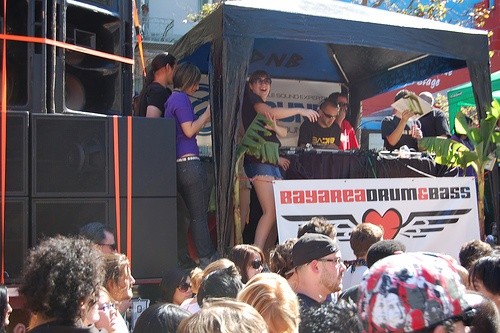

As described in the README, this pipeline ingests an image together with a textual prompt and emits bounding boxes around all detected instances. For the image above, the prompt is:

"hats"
[285,232,339,275]
[357,251,482,333]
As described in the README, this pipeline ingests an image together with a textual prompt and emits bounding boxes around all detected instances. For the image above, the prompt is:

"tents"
[164,0,500,257]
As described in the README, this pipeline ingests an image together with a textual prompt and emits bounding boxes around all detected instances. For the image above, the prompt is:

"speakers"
[0,0,178,283]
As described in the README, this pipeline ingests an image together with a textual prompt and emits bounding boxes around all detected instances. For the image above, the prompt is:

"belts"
[176,155,201,162]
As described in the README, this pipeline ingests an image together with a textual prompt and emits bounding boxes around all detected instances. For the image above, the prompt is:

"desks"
[242,146,436,245]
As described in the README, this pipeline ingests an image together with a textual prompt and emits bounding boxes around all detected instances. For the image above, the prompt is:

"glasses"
[251,259,262,268]
[97,242,118,251]
[336,102,350,107]
[316,255,342,265]
[254,78,272,84]
[322,108,339,119]
[446,308,476,325]
[98,302,116,311]
[177,282,190,292]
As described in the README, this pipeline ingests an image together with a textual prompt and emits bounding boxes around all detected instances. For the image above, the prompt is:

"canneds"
[411,125,418,138]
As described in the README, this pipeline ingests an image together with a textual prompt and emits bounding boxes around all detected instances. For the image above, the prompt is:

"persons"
[298,101,344,150]
[447,116,478,186]
[417,92,453,138]
[380,89,426,150]
[243,71,320,259]
[325,92,359,150]
[0,218,500,333]
[164,62,216,265]
[138,53,179,118]
